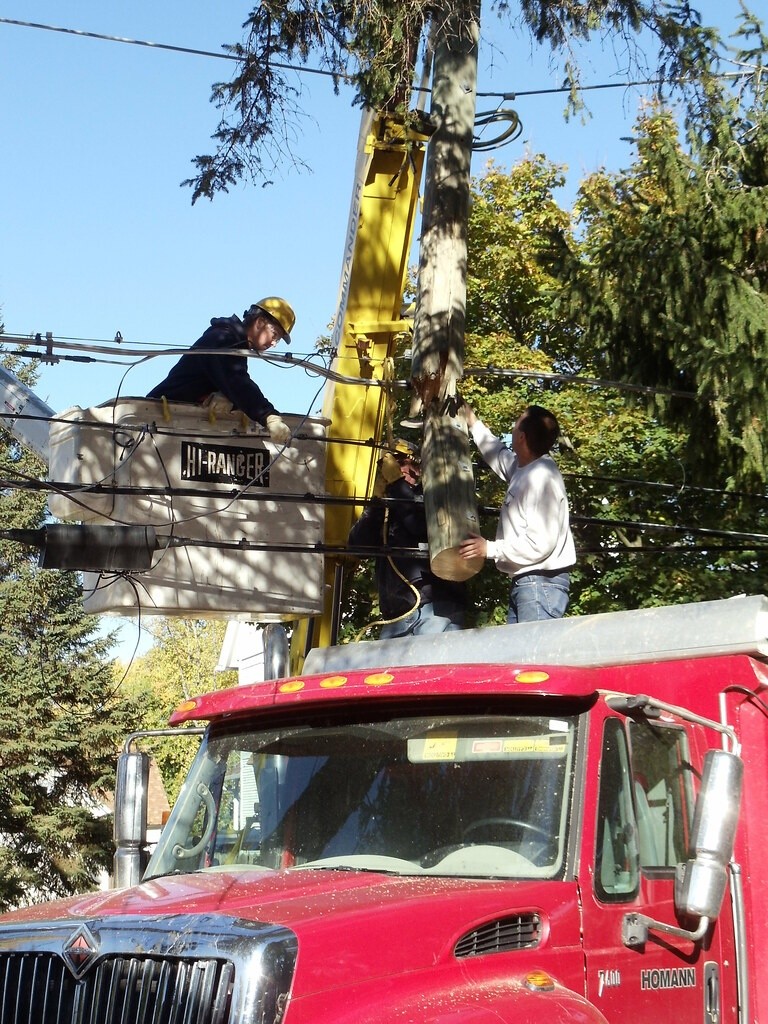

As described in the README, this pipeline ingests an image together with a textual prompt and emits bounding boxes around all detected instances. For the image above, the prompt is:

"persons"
[145,296,296,445]
[346,438,467,640]
[459,401,577,624]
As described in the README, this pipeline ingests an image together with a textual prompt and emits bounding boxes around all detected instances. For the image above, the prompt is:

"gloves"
[267,414,292,448]
[373,473,389,503]
[382,453,406,484]
[203,390,233,424]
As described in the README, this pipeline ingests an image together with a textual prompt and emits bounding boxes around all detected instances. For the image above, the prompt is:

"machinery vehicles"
[0,0,768,1024]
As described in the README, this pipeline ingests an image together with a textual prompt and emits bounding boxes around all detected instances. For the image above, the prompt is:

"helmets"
[251,296,295,344]
[377,438,420,466]
